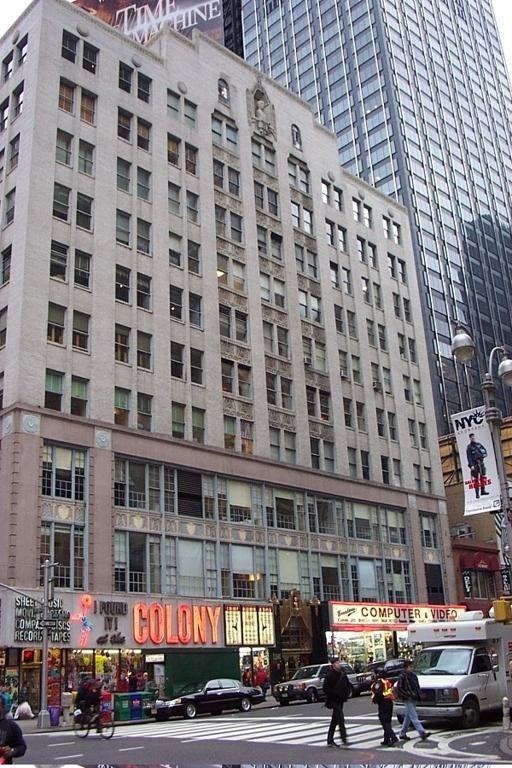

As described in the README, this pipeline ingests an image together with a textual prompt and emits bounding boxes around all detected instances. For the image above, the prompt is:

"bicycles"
[72,703,116,741]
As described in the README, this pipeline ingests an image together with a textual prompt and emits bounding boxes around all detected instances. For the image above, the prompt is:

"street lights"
[449,321,512,599]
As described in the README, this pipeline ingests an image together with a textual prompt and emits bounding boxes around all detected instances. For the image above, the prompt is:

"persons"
[322,657,353,746]
[0,680,15,720]
[243,667,252,686]
[467,432,489,498]
[397,661,431,741]
[257,668,268,695]
[0,694,27,764]
[75,672,104,732]
[371,664,400,747]
[13,678,37,719]
[116,669,149,691]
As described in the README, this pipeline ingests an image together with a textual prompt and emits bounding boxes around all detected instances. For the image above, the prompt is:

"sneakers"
[399,735,411,741]
[341,738,352,745]
[380,740,391,745]
[422,732,432,740]
[327,741,340,749]
[388,738,398,747]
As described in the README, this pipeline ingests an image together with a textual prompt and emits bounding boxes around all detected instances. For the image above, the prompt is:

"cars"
[143,677,266,720]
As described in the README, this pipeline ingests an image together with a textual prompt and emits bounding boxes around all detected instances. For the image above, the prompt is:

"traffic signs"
[35,618,59,627]
[51,613,70,621]
[32,608,44,614]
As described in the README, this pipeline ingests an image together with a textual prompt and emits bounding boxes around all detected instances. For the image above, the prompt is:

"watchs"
[10,746,18,756]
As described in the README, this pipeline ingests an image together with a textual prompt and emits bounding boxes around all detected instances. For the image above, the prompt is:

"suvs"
[356,656,412,694]
[271,661,360,706]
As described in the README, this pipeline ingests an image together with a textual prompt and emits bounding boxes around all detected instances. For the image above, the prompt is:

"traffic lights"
[23,650,36,662]
[493,595,512,624]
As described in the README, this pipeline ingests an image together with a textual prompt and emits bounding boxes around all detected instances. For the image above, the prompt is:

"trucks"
[391,610,512,729]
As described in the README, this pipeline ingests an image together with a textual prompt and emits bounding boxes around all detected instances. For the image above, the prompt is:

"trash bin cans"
[47,705,61,727]
[100,691,153,723]
